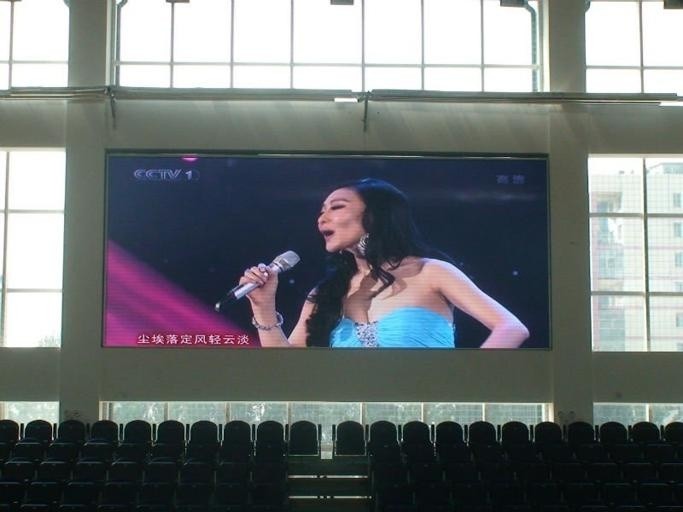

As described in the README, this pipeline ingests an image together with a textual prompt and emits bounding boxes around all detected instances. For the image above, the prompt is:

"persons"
[238,178,530,350]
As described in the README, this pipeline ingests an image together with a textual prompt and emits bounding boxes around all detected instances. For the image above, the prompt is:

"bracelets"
[250,310,285,331]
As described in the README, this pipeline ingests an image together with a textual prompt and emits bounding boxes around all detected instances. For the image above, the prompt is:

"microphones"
[215,250,301,313]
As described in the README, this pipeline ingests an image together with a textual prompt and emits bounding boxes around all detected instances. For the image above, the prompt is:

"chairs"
[1,416,288,510]
[288,420,322,500]
[369,421,683,511]
[331,420,369,497]
